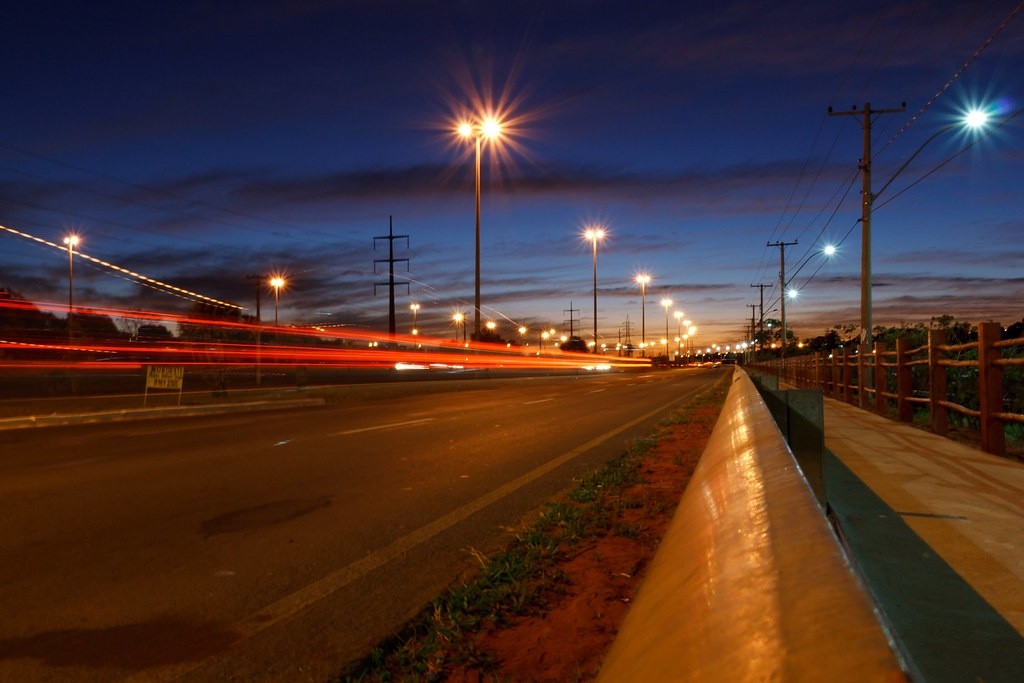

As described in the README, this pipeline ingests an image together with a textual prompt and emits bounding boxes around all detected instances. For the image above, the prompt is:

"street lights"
[457,113,503,342]
[410,303,421,350]
[269,277,285,345]
[760,288,799,361]
[857,108,991,402]
[453,313,463,340]
[675,311,685,367]
[661,298,672,364]
[62,234,79,340]
[751,308,779,362]
[584,227,604,354]
[636,273,650,359]
[778,244,837,383]
